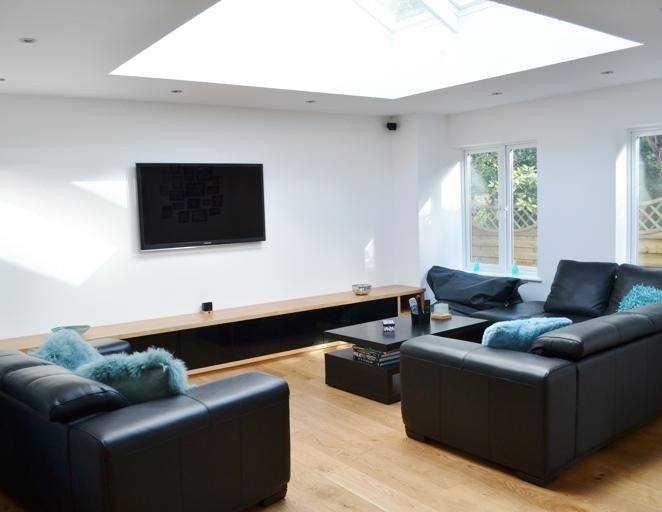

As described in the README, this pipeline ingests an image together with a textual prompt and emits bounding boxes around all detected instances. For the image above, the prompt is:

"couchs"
[400,264,661,486]
[0,339,290,512]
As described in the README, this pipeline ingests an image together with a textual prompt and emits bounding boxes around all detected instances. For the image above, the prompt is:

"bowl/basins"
[351,284,372,296]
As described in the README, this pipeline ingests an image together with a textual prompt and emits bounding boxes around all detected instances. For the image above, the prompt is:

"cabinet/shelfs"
[0,284,427,378]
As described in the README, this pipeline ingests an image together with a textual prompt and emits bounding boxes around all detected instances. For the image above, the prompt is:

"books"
[352,344,400,367]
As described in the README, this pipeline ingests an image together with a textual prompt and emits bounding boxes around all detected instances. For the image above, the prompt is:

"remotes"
[424,300,429,313]
[409,295,418,315]
[416,295,423,314]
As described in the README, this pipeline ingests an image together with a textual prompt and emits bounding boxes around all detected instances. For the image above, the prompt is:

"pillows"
[31,327,104,377]
[615,282,661,312]
[541,255,619,315]
[481,316,572,349]
[70,347,197,403]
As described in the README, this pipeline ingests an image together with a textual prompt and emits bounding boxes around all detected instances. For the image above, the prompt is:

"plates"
[51,326,89,335]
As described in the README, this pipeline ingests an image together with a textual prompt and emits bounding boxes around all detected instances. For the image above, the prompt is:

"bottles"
[473,257,480,271]
[512,261,519,276]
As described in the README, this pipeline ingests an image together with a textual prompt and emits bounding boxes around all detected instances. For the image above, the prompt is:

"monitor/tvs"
[136,163,265,250]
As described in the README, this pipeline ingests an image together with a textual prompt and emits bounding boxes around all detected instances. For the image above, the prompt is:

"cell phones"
[382,319,395,326]
